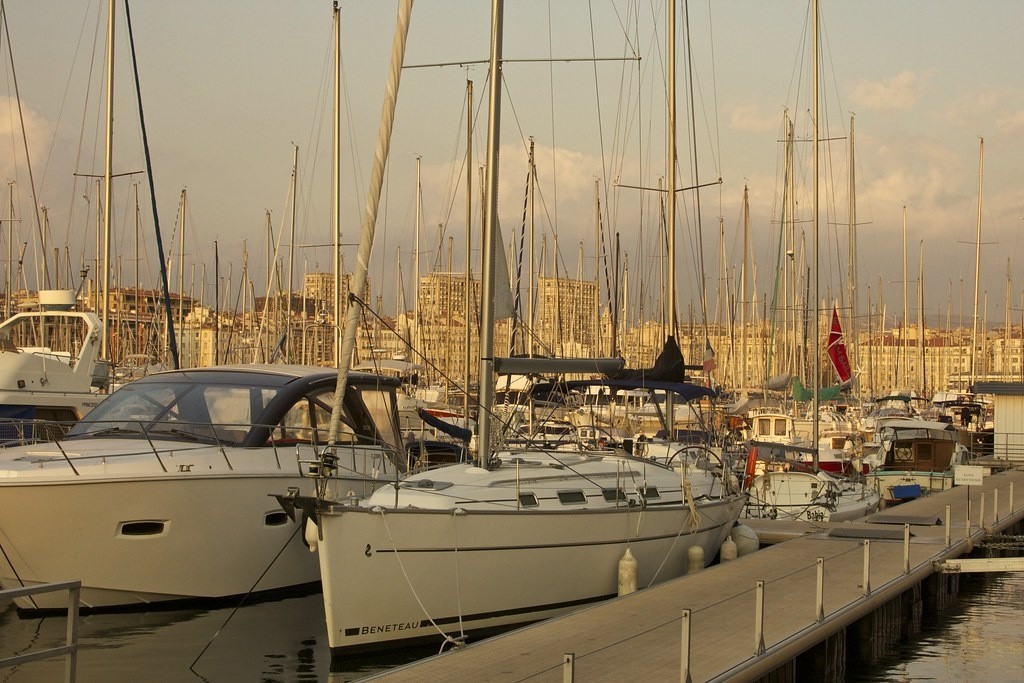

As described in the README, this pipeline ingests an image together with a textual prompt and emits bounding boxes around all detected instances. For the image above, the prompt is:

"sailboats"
[0,0,1021,674]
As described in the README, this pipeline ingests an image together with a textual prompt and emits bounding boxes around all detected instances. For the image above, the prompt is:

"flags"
[703,332,718,374]
[825,303,853,384]
